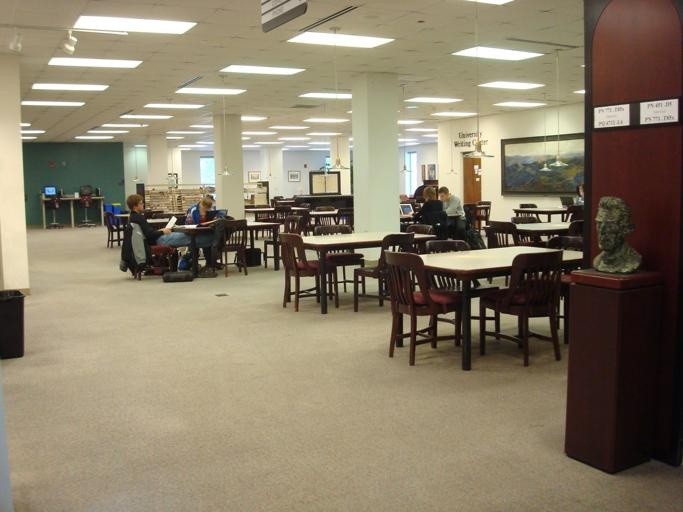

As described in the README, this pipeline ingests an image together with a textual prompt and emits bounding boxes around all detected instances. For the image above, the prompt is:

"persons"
[127,194,192,248]
[412,187,444,226]
[594,195,643,273]
[178,195,226,271]
[438,185,466,239]
[400,183,426,215]
[574,184,584,205]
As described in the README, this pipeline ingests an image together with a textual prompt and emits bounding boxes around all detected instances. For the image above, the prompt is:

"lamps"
[399,83,410,173]
[129,147,140,182]
[1,23,129,57]
[215,96,239,177]
[461,3,499,158]
[168,146,177,187]
[538,47,570,173]
[329,25,351,171]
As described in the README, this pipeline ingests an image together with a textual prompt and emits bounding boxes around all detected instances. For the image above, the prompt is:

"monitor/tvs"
[44,185,58,197]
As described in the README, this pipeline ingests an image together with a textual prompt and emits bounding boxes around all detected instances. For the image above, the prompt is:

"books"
[164,216,178,229]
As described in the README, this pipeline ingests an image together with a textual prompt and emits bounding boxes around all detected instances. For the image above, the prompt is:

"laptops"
[204,209,228,224]
[400,202,414,216]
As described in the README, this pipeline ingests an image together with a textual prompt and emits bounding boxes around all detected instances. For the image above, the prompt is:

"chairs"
[47,196,62,228]
[79,194,97,227]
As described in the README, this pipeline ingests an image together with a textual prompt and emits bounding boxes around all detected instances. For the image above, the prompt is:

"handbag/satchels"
[236,248,262,268]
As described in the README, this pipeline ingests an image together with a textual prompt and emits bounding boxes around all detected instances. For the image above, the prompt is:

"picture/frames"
[500,132,585,198]
[428,164,436,180]
[289,171,300,182]
[248,172,261,182]
[421,164,426,179]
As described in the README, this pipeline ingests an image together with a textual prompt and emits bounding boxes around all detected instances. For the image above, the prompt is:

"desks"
[38,189,105,229]
[294,194,354,207]
